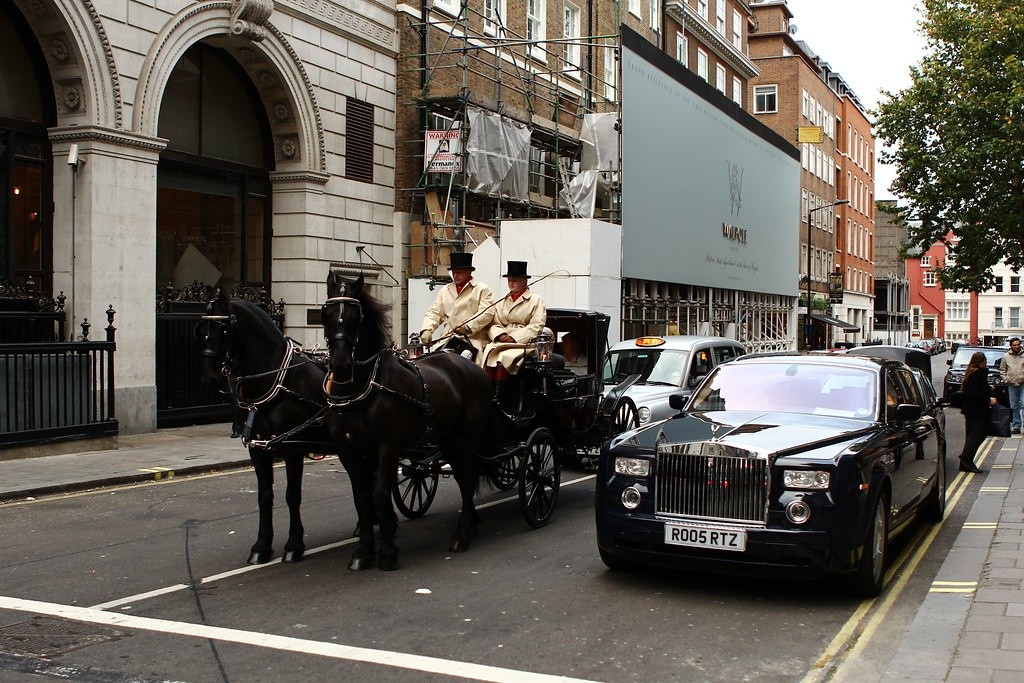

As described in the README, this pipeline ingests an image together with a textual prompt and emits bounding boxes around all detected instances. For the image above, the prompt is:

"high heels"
[959,460,982,473]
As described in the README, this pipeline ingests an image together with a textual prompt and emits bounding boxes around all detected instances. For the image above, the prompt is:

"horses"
[321,270,500,571]
[194,285,379,565]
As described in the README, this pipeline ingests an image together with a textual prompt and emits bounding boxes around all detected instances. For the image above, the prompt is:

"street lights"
[808,200,850,345]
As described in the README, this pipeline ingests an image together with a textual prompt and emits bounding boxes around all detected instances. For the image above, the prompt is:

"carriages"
[196,272,640,571]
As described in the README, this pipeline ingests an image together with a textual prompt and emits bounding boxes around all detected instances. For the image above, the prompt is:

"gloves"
[420,330,432,346]
[454,324,466,335]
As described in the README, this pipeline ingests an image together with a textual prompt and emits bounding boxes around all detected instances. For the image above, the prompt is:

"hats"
[503,261,531,278]
[447,253,475,270]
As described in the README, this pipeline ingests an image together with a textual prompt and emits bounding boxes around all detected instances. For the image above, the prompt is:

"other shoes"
[1012,429,1020,434]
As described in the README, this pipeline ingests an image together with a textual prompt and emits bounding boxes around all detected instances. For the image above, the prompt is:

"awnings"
[810,314,861,333]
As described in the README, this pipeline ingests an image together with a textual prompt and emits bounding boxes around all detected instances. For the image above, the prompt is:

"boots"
[491,381,505,405]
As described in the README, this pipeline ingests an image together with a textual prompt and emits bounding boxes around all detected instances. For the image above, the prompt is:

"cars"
[553,335,747,467]
[595,345,947,598]
[944,346,1010,404]
[951,339,970,354]
[905,337,947,356]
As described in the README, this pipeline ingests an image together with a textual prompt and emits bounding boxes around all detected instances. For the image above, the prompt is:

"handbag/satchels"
[987,404,1011,437]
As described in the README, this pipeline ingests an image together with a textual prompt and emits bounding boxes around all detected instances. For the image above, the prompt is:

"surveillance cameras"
[68,144,79,166]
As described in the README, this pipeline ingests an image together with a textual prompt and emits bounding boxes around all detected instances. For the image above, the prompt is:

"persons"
[959,352,998,474]
[539,327,588,376]
[420,252,547,408]
[999,339,1024,434]
